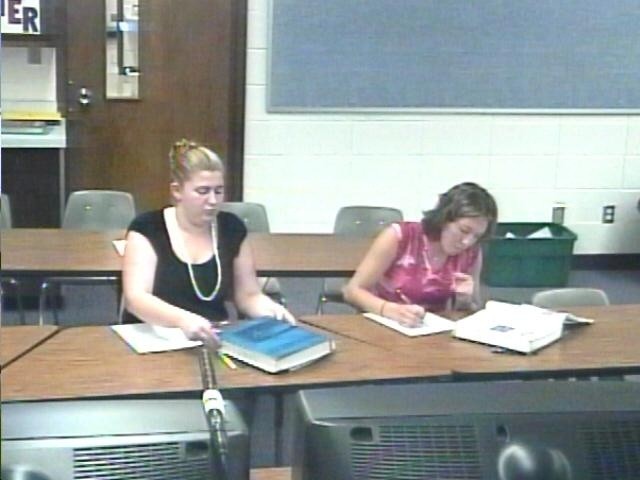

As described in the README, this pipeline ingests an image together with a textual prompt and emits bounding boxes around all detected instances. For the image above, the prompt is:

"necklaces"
[183,219,224,302]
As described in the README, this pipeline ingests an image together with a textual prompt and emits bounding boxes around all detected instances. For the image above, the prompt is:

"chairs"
[221,201,284,308]
[531,288,608,305]
[315,206,403,316]
[38,189,135,326]
[0,194,24,326]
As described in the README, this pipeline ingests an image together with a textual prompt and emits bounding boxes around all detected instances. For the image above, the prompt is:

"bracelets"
[381,300,388,316]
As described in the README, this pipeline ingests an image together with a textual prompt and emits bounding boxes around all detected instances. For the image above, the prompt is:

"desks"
[1,228,374,273]
[0,323,62,370]
[450,305,640,375]
[1,315,449,401]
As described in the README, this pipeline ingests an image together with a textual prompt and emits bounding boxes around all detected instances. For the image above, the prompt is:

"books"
[109,323,204,354]
[1,119,47,135]
[451,300,595,354]
[214,317,334,374]
[362,312,456,337]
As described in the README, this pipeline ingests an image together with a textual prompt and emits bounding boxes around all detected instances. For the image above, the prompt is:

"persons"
[121,139,298,350]
[343,181,498,328]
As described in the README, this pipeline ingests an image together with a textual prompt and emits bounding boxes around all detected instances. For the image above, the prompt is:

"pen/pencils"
[218,351,237,368]
[395,289,427,321]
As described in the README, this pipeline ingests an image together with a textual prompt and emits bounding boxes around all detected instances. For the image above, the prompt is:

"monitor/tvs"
[0,398,250,480]
[291,381,639,480]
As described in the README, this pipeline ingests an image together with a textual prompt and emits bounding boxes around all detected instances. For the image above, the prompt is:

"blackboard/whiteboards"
[264,0,639,112]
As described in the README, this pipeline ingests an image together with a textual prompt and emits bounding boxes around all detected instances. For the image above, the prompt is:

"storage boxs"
[483,222,577,286]
